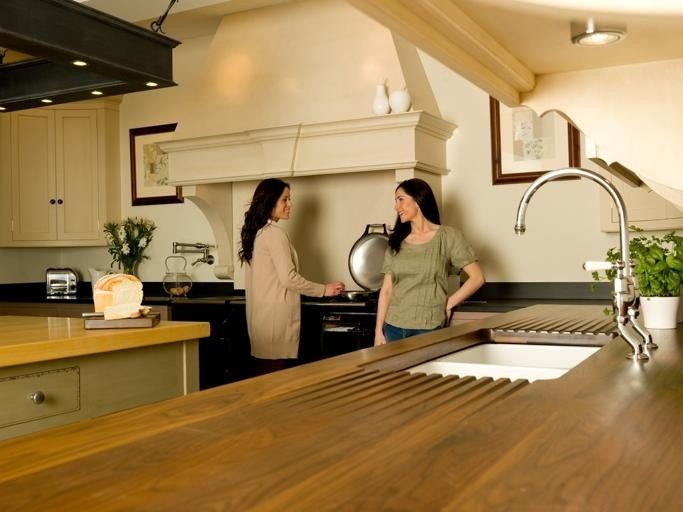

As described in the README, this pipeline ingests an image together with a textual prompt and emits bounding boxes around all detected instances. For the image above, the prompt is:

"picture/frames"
[128,123,188,207]
[487,88,583,186]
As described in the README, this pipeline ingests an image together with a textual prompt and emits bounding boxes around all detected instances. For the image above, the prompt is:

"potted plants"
[585,227,682,330]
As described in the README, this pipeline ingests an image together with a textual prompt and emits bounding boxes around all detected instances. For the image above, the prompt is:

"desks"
[0,310,210,442]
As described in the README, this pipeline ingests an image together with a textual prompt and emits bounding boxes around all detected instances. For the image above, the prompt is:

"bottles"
[372,83,412,114]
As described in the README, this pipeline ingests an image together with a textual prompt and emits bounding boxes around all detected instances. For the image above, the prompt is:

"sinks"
[357,326,617,380]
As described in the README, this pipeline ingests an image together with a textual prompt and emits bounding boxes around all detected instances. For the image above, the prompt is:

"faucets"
[514,167,658,361]
[172,242,216,264]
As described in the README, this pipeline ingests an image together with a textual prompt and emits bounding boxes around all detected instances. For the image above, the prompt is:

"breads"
[92,274,151,320]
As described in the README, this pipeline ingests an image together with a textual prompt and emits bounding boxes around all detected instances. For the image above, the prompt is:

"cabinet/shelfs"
[174,304,318,389]
[0,96,120,250]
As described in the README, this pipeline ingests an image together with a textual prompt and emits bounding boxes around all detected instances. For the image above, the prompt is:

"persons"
[372,178,486,348]
[237,178,345,378]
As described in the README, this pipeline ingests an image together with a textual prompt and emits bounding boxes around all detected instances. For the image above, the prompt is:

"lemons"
[170,286,190,295]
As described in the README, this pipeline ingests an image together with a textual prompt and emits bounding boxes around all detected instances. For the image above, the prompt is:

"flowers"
[96,213,157,275]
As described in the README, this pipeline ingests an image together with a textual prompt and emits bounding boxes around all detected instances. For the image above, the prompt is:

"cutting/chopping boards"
[84,311,162,330]
